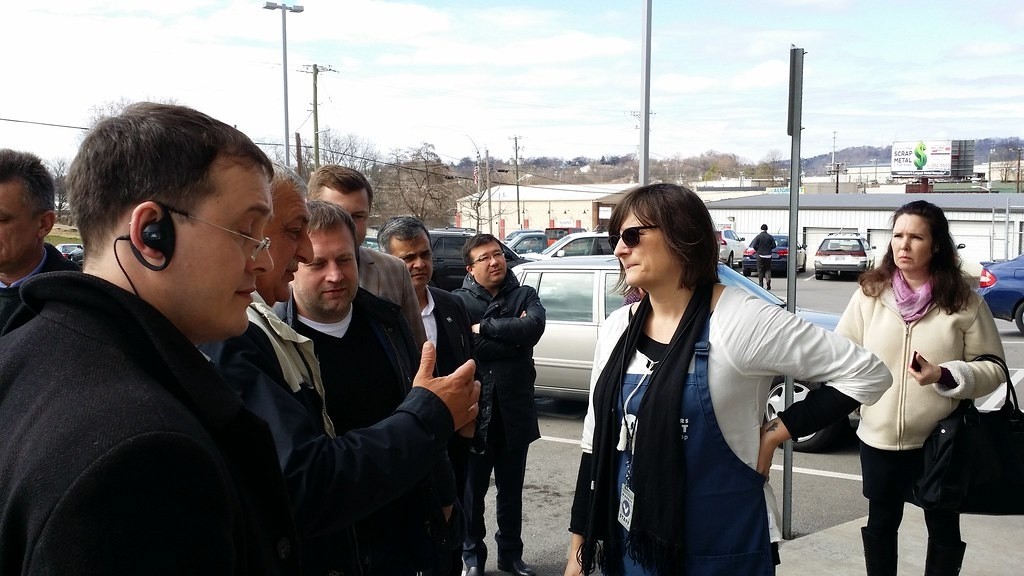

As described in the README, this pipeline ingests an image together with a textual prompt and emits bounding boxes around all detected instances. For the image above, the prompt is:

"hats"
[761,223,768,230]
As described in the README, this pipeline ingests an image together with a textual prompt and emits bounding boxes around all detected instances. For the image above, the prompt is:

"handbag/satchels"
[911,354,1024,515]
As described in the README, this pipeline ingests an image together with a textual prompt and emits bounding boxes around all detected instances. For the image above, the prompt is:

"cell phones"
[910,351,927,372]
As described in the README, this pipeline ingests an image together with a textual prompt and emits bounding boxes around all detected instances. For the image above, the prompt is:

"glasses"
[607,225,659,248]
[128,201,272,259]
[469,250,505,266]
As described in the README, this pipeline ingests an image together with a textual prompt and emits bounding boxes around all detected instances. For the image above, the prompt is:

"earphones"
[113,199,178,299]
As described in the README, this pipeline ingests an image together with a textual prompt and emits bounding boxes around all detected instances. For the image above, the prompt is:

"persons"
[189,157,483,576]
[562,185,893,576]
[267,200,469,576]
[304,165,439,384]
[446,231,547,576]
[754,225,777,290]
[378,214,476,511]
[0,148,85,331]
[1,100,304,574]
[831,200,1008,576]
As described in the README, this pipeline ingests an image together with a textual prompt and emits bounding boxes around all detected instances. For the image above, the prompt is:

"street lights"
[263,2,304,166]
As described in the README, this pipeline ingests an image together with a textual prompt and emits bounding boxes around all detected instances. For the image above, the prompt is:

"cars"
[948,230,965,268]
[511,255,858,453]
[716,229,748,270]
[976,252,1024,333]
[56,243,85,270]
[742,226,807,278]
[814,227,876,281]
[355,225,613,295]
